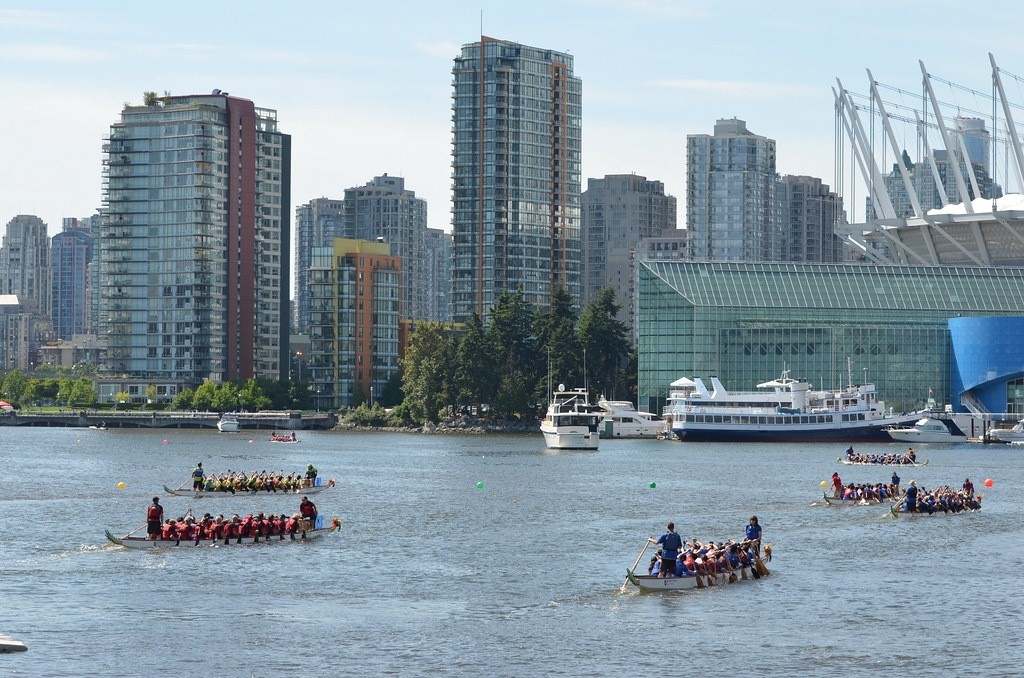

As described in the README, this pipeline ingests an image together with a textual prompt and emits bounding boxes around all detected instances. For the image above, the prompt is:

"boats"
[270,438,301,444]
[217,414,240,432]
[662,357,1024,445]
[823,492,981,517]
[837,457,928,467]
[162,478,335,496]
[89,426,109,431]
[627,544,771,590]
[105,518,342,547]
[540,347,680,448]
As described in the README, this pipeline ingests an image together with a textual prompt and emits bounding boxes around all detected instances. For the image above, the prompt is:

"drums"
[301,479,310,489]
[299,518,308,532]
[850,454,855,461]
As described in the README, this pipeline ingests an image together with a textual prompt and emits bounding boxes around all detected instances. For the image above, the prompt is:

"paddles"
[843,482,897,506]
[167,476,193,494]
[683,537,770,589]
[212,469,296,494]
[905,455,918,467]
[887,496,904,514]
[816,488,832,502]
[167,508,308,546]
[620,534,652,589]
[105,522,148,546]
[852,454,904,466]
[917,484,981,515]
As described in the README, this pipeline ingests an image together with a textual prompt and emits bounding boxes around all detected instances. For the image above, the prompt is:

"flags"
[929,388,933,394]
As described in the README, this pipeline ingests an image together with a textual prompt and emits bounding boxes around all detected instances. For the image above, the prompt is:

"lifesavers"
[842,405,847,411]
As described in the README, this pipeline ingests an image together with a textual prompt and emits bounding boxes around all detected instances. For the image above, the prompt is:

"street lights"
[369,386,374,407]
[83,338,91,374]
[5,392,15,404]
[297,351,302,381]
[317,390,321,413]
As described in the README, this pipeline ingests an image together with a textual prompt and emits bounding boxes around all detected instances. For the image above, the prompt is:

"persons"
[845,446,915,464]
[194,409,196,417]
[648,514,762,578]
[901,478,974,513]
[271,431,296,441]
[832,471,900,500]
[147,496,318,541]
[102,421,106,426]
[192,462,317,494]
